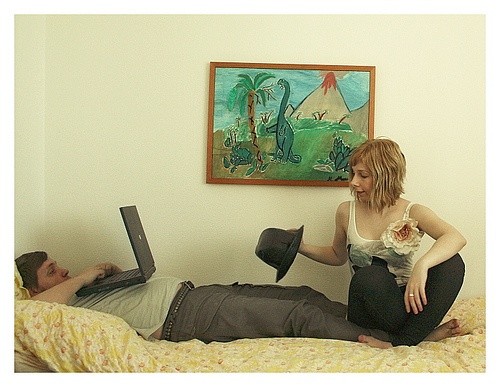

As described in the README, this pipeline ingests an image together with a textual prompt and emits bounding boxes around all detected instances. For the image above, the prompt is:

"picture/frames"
[205,60,376,188]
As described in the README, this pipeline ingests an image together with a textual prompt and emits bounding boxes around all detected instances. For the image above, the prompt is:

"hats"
[254,223,304,284]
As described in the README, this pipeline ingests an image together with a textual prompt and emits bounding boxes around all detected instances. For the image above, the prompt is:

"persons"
[13,251,394,345]
[286,137,467,350]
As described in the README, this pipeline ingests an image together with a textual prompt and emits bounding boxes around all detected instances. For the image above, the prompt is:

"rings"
[408,294,414,296]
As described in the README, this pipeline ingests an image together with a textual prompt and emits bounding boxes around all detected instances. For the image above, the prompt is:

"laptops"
[75,206,156,296]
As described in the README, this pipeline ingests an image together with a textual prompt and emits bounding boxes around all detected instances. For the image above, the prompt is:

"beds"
[13,260,484,371]
